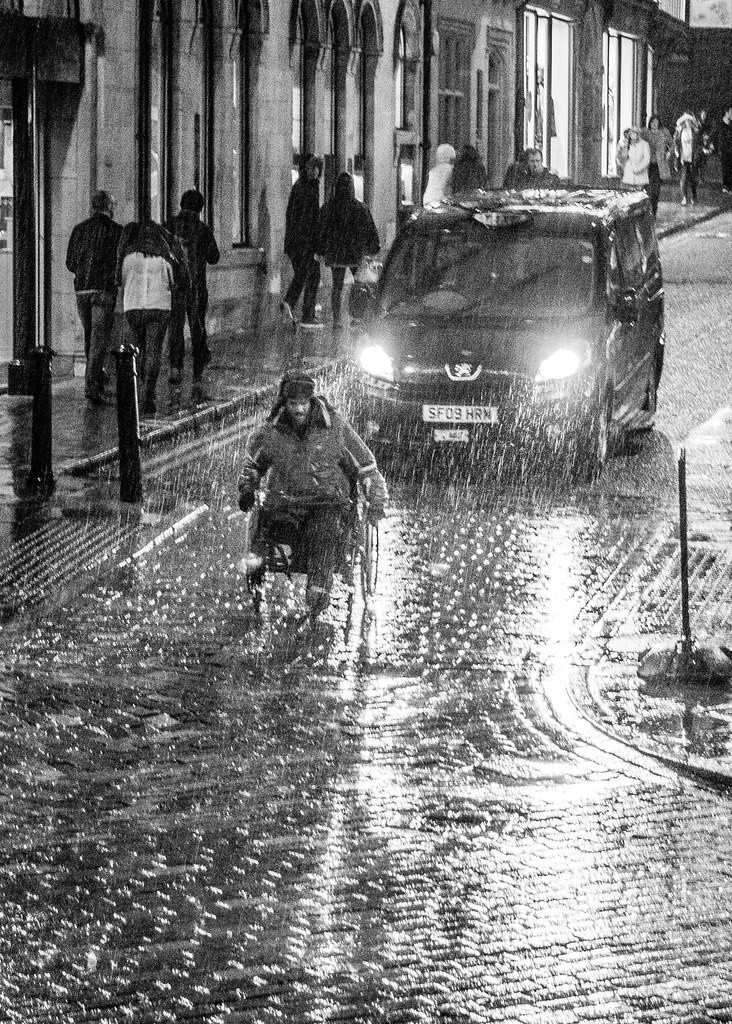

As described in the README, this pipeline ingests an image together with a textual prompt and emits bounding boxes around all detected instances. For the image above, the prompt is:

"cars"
[346,185,666,487]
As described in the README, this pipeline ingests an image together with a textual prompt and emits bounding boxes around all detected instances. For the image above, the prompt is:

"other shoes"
[281,302,292,322]
[143,396,155,413]
[309,589,331,613]
[86,390,103,403]
[300,319,323,329]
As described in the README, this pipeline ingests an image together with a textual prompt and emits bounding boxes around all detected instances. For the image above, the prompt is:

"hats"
[267,370,331,429]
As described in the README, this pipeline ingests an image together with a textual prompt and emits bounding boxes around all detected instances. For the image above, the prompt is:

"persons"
[63,189,122,406]
[672,104,732,207]
[161,190,221,401]
[115,218,193,412]
[279,150,328,329]
[315,169,381,332]
[616,114,678,223]
[502,148,561,189]
[238,371,387,611]
[420,142,486,210]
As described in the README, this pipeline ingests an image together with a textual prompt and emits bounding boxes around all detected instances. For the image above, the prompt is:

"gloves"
[239,492,255,512]
[366,504,384,525]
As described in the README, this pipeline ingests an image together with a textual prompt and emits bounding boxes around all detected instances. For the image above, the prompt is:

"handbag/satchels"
[349,259,377,317]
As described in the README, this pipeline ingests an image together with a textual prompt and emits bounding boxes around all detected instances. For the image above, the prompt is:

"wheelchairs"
[242,489,381,607]
